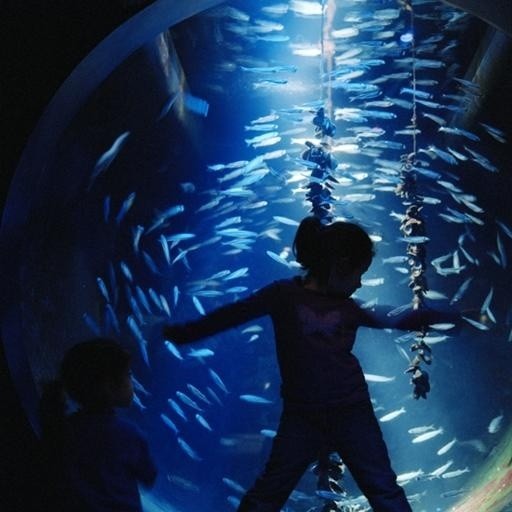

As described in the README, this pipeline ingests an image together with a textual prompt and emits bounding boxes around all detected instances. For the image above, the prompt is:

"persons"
[157,217,484,512]
[43,339,154,512]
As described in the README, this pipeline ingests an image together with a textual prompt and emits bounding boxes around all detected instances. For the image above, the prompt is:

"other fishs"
[83,0,512,512]
